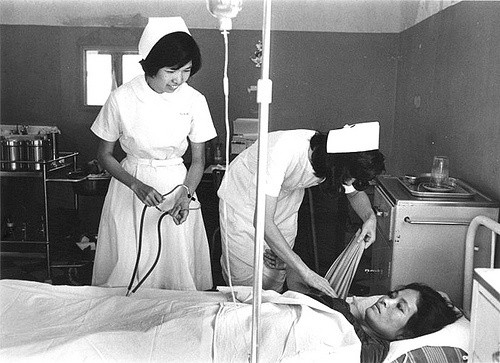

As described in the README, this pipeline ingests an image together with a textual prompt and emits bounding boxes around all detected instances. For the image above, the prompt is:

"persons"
[91,17,217,293]
[218,121,386,296]
[302,282,456,363]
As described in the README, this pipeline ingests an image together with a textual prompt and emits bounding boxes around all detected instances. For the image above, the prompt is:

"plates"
[423,183,456,192]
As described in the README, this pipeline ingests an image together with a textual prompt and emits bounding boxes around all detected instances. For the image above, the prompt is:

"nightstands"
[468,268,500,363]
[369,177,499,309]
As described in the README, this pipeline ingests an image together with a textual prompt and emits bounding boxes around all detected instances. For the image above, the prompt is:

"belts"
[128,155,184,167]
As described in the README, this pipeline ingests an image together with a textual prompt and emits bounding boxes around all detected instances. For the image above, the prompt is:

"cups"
[430,155,449,189]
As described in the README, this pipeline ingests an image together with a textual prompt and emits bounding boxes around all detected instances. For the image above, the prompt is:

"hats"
[138,16,192,60]
[327,121,380,153]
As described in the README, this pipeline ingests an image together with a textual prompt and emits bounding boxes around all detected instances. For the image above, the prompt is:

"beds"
[0,215,500,363]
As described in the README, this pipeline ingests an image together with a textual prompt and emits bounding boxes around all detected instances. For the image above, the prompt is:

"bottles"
[204,135,224,165]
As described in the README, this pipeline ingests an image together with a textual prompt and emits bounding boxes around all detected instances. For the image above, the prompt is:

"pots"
[0,135,51,171]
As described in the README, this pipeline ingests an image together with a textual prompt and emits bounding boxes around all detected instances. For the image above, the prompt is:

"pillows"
[351,295,469,363]
